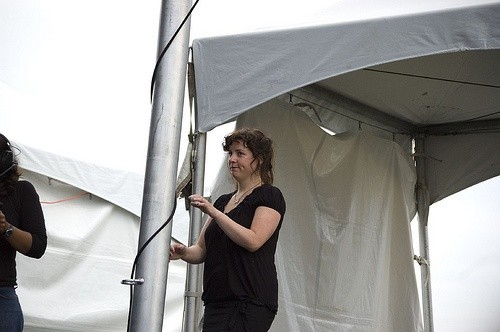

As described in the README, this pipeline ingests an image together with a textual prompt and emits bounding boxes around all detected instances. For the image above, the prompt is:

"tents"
[0,138,192,332]
[186,1,500,331]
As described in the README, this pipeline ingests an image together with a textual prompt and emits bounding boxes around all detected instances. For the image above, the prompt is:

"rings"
[202,197,205,203]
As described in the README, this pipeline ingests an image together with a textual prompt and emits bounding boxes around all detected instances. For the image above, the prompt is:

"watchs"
[2,223,14,237]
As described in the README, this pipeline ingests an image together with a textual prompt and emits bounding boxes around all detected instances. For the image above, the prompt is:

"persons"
[0,133,48,332]
[168,127,287,332]
